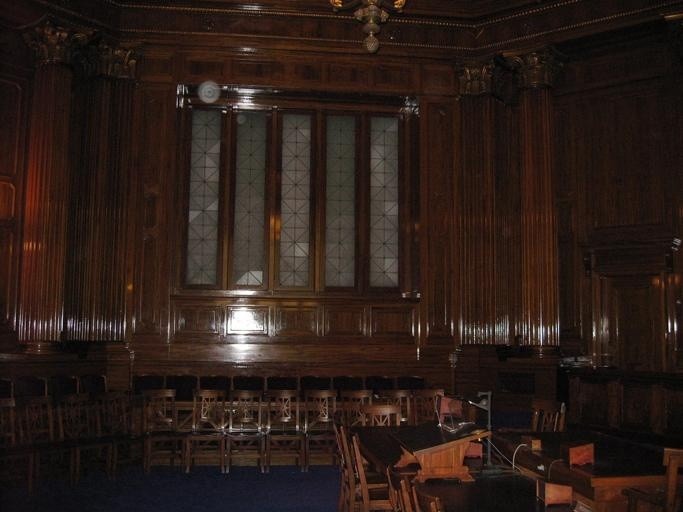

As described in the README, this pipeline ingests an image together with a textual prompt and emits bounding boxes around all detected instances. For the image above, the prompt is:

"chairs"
[334,390,683,512]
[186,391,226,474]
[305,390,338,470]
[146,391,186,474]
[266,391,304,472]
[226,391,265,473]
[2,390,146,494]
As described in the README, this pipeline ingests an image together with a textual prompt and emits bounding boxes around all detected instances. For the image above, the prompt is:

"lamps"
[331,0,407,53]
